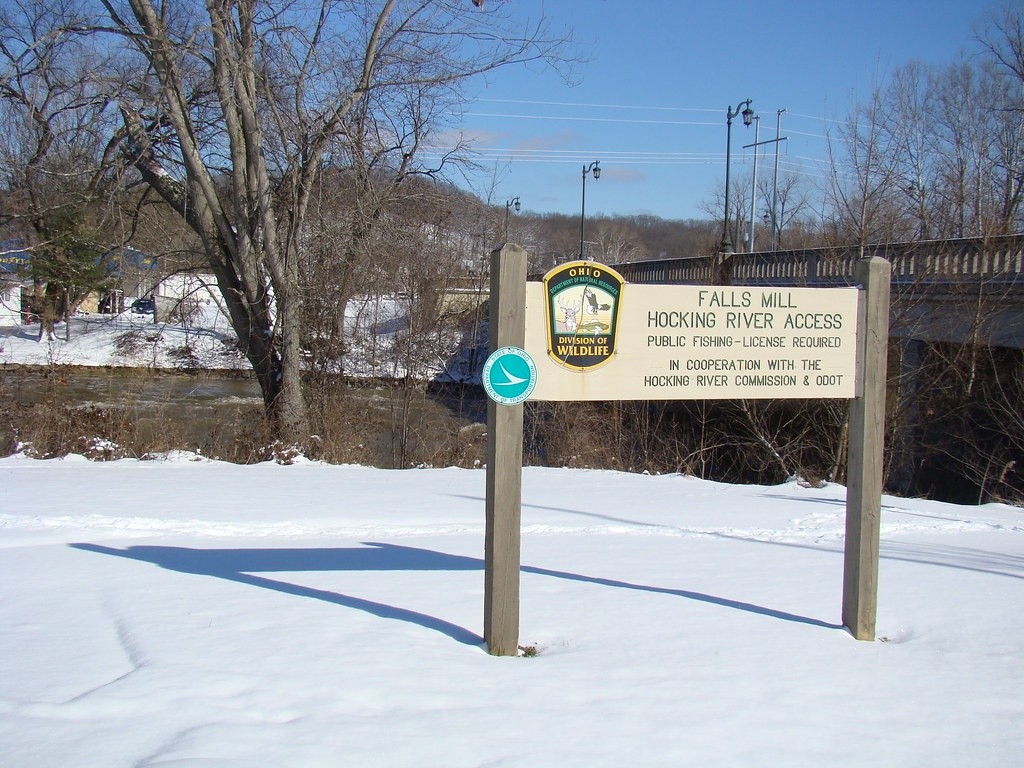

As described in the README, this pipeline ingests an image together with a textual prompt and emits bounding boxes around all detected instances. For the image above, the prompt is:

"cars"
[131,298,154,314]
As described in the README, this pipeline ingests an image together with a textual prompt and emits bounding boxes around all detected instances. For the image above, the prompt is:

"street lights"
[720,99,754,253]
[506,196,521,243]
[579,160,601,260]
